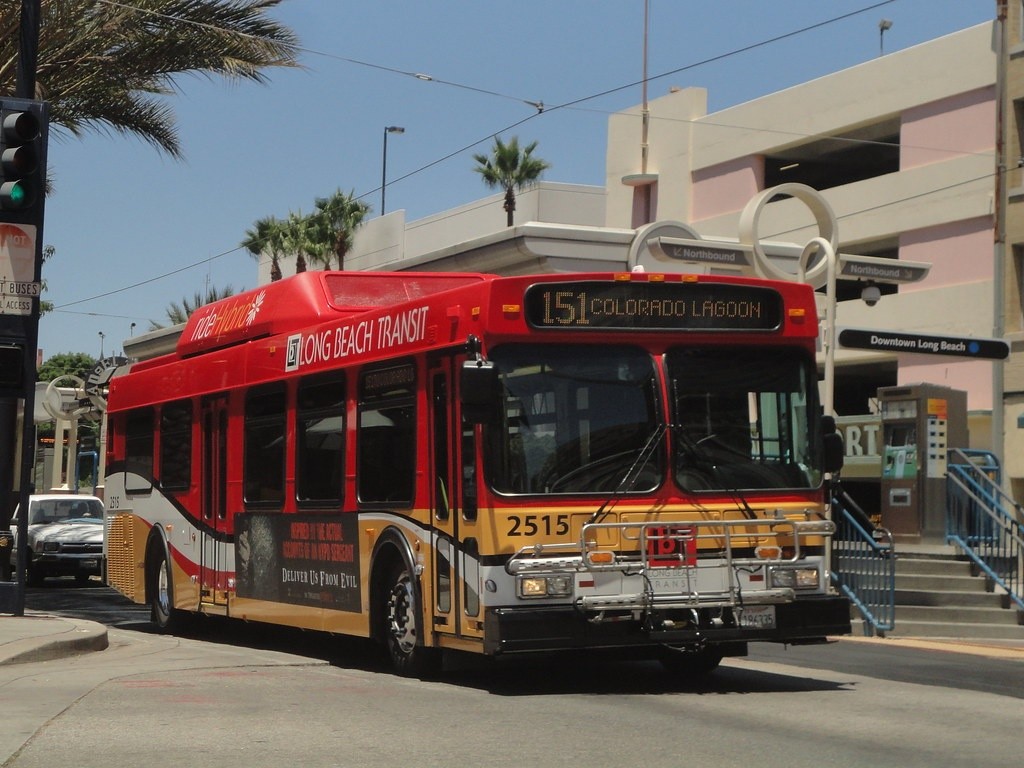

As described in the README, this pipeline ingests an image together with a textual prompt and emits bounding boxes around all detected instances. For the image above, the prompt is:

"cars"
[9,492,105,586]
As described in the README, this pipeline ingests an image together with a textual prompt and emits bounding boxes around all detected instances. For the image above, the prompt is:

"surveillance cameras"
[861,287,881,306]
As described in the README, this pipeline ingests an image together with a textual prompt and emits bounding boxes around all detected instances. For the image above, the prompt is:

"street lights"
[381,125,404,214]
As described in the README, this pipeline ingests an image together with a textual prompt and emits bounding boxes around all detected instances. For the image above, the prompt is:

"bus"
[97,269,854,683]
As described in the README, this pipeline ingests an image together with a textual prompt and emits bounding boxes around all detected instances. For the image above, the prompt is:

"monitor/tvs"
[891,429,908,447]
